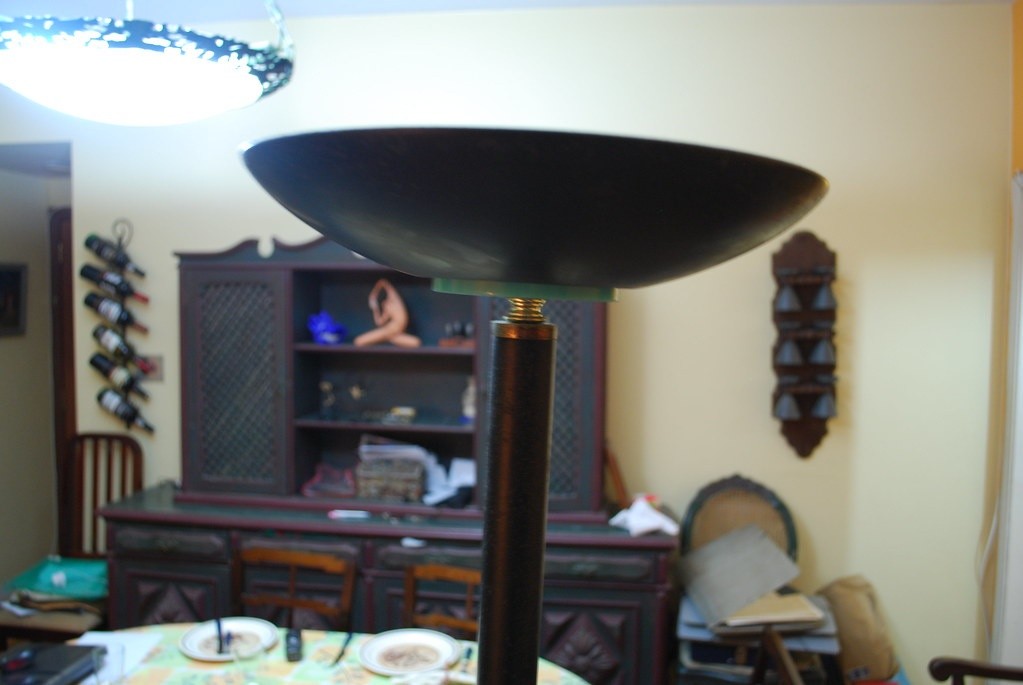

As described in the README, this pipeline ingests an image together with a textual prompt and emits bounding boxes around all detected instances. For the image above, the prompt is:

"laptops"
[0,641,108,685]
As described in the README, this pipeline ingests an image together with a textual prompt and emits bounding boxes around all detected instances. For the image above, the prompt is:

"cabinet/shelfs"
[105,232,676,685]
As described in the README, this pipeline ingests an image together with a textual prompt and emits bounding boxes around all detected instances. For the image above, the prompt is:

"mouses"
[0,647,37,674]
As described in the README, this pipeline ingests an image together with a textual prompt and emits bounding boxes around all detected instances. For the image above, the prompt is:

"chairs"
[403,563,481,641]
[229,530,358,632]
[1,431,145,655]
[677,472,833,683]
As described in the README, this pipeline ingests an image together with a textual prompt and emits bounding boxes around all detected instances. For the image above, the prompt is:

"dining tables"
[69,618,588,685]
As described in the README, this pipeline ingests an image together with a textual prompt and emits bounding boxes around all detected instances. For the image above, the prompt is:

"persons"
[353,277,421,348]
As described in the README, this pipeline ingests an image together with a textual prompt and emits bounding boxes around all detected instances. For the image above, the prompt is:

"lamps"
[0,1,294,125]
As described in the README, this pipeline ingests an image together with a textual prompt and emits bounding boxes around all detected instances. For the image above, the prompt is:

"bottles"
[84,235,145,278]
[89,354,149,402]
[92,323,153,373]
[96,388,153,434]
[80,265,149,303]
[84,293,147,333]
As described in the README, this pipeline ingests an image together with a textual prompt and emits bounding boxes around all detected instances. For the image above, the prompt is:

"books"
[676,521,827,636]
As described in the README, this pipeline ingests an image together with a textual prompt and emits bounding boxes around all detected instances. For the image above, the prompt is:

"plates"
[356,627,462,676]
[178,616,280,662]
